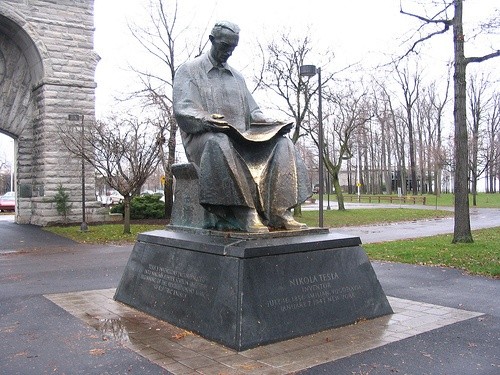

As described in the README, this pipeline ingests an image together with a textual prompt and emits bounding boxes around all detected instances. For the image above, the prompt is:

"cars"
[0,190,15,212]
[104,189,166,204]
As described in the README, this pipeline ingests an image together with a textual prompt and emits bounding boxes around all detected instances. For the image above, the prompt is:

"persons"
[172,21,314,233]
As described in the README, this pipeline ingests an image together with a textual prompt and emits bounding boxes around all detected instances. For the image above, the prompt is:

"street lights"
[67,113,89,230]
[300,64,323,227]
